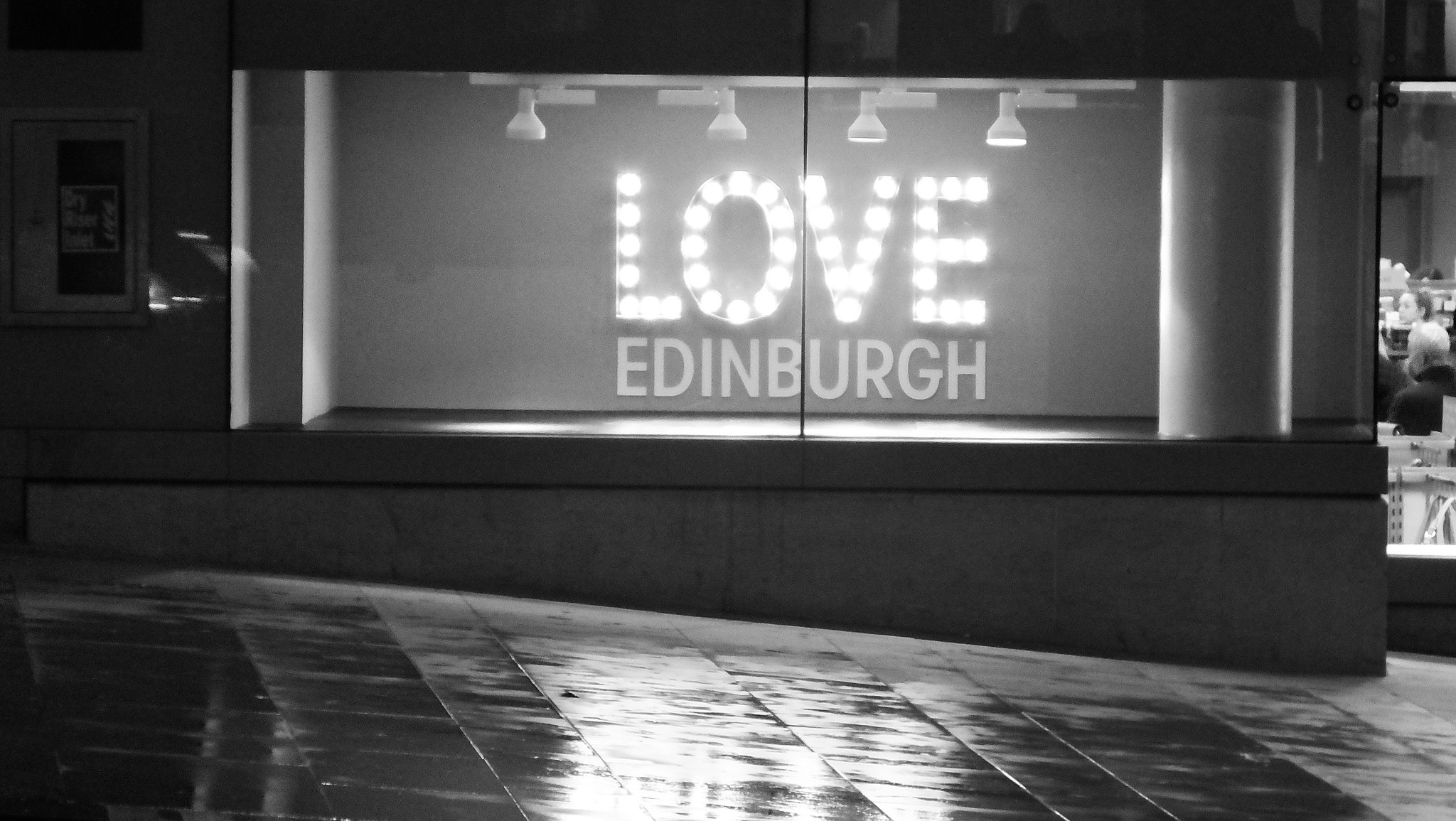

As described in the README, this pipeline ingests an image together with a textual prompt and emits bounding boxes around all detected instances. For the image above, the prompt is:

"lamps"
[847,88,937,143]
[657,87,747,139]
[985,88,1078,147]
[505,85,595,140]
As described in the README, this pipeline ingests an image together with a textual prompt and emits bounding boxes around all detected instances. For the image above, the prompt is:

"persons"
[1387,321,1455,436]
[1377,353,1412,421]
[1410,266,1442,281]
[1397,288,1434,324]
